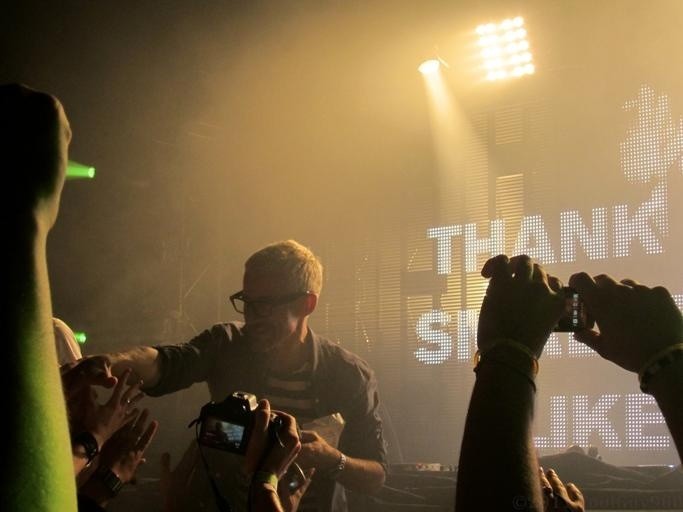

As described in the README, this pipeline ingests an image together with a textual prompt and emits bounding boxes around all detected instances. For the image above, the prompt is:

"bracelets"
[473,340,539,375]
[472,361,536,391]
[639,338,682,394]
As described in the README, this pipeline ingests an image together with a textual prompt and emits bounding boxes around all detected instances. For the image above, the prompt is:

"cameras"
[551,286,596,333]
[197,389,282,457]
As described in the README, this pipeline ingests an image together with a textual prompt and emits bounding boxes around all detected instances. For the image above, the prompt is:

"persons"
[62,239,389,512]
[62,371,314,512]
[540,465,584,511]
[0,84,77,511]
[454,254,681,511]
[53,319,83,364]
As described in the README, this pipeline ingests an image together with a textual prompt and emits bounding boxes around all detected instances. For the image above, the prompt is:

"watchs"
[316,454,346,481]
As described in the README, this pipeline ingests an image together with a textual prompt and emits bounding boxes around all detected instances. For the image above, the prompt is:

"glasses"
[230,289,312,317]
